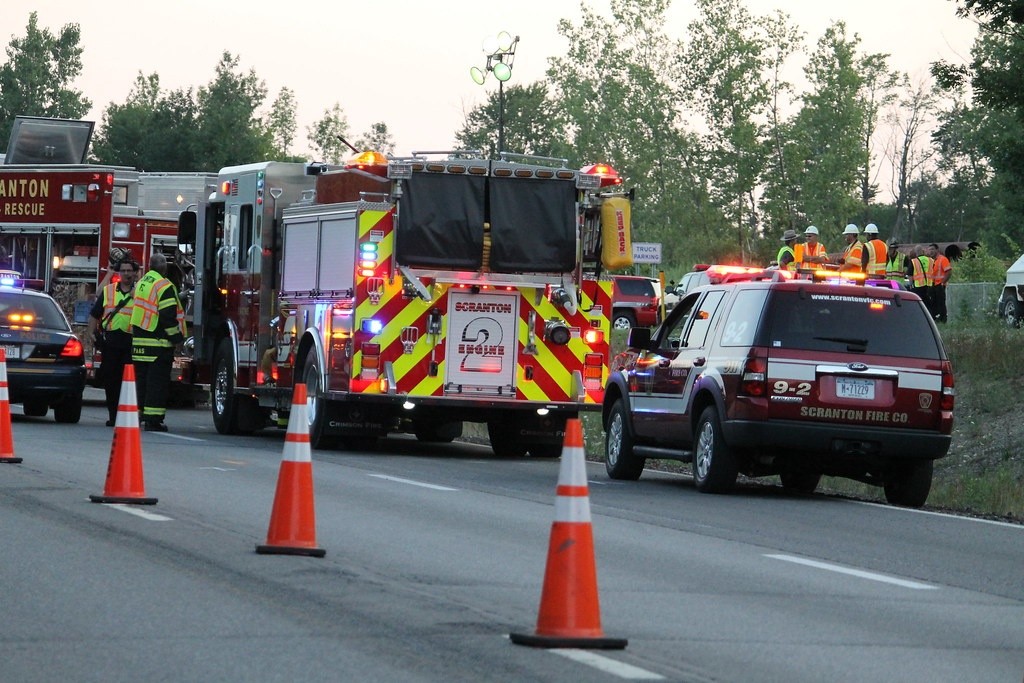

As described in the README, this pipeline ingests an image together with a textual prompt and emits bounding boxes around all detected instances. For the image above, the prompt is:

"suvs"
[600,268,955,508]
[607,274,661,330]
[658,263,742,325]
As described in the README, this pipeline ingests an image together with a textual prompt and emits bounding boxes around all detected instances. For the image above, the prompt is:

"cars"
[998,255,1024,329]
[0,269,86,423]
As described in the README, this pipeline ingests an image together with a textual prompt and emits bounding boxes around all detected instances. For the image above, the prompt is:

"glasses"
[806,234,815,238]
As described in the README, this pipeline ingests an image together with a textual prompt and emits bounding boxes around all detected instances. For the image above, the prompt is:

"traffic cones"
[89,364,159,505]
[255,382,326,558]
[0,349,23,463]
[509,420,629,651]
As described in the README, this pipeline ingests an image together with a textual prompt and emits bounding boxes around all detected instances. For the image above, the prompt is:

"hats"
[780,230,801,241]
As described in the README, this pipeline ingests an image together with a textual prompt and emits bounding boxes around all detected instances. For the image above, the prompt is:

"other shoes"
[144,421,168,431]
[106,408,117,426]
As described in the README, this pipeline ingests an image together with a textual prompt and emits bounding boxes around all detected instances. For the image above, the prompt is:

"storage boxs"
[75,245,91,256]
[91,246,98,256]
[77,284,96,300]
[73,301,95,323]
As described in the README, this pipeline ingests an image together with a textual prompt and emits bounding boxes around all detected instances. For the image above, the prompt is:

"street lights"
[470,32,521,158]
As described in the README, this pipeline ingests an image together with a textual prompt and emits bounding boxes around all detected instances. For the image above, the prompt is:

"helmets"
[863,223,879,233]
[841,224,860,234]
[804,226,819,235]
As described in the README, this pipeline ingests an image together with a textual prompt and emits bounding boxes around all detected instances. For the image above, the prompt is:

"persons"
[777,225,829,270]
[886,243,952,323]
[87,261,137,426]
[838,223,891,279]
[131,254,188,431]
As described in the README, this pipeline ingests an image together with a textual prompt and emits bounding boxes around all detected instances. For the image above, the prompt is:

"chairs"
[811,307,837,335]
[856,304,909,340]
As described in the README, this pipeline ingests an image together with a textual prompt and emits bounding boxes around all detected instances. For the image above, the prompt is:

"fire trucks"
[177,150,636,460]
[0,114,218,410]
